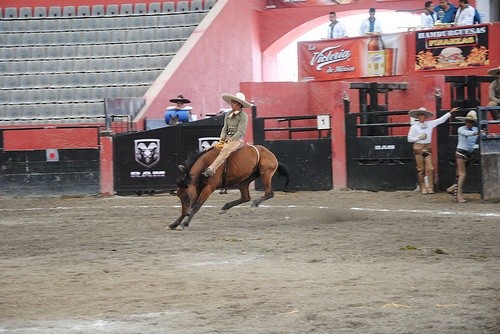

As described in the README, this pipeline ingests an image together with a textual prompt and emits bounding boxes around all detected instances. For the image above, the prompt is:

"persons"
[453,0,480,24]
[360,8,382,35]
[418,1,438,26]
[447,110,485,203]
[166,95,193,111]
[431,0,457,23]
[487,66,500,125]
[325,12,348,38]
[202,92,253,177]
[407,106,459,194]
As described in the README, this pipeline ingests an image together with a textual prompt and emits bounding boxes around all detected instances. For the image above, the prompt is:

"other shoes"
[454,195,467,203]
[446,184,457,192]
[420,188,434,194]
[202,168,214,177]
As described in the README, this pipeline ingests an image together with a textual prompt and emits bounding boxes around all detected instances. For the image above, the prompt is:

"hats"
[487,66,500,74]
[455,110,478,121]
[408,106,434,117]
[169,95,191,104]
[222,92,253,108]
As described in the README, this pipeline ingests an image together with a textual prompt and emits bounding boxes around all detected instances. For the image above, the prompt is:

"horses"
[163,142,292,231]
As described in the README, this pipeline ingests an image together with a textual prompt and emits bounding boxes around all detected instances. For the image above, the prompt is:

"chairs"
[0,0,216,123]
